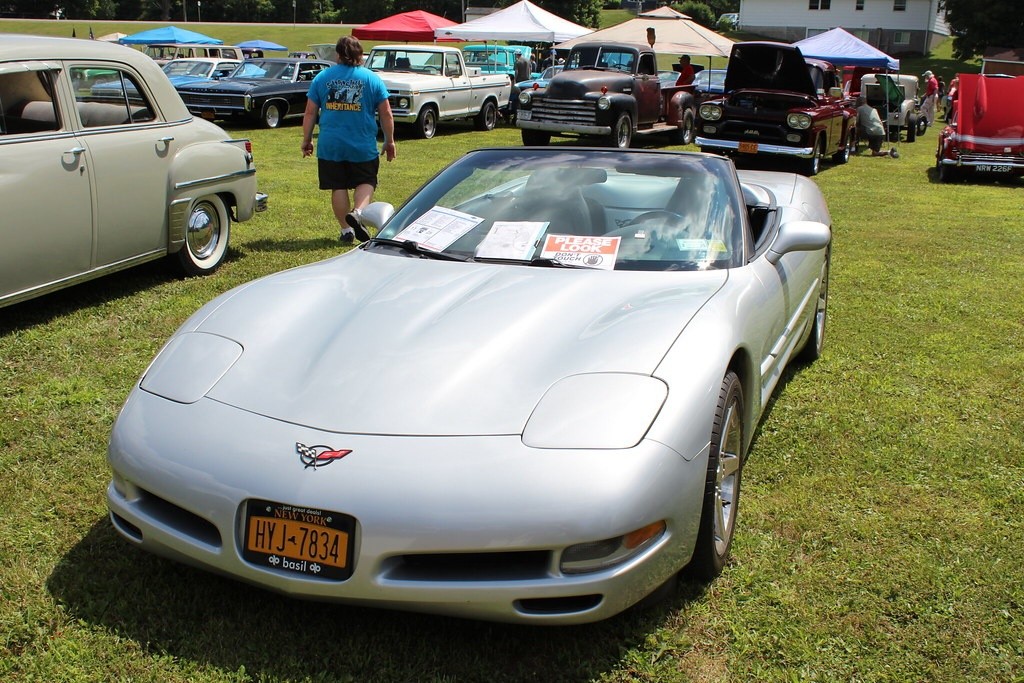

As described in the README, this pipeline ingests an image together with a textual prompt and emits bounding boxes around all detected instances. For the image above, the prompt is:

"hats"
[551,50,557,55]
[922,70,933,76]
[678,55,690,60]
[513,49,522,54]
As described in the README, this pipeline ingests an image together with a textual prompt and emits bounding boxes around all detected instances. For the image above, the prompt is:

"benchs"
[6,99,147,127]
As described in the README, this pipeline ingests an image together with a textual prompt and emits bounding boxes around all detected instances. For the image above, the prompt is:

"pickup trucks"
[364,45,512,139]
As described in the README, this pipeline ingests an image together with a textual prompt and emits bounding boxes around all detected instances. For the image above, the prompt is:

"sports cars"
[107,146,832,625]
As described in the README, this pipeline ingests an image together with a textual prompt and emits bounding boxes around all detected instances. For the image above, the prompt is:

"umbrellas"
[548,5,734,57]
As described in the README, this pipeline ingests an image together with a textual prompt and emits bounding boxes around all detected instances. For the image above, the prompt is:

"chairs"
[519,170,592,238]
[665,177,727,239]
[392,58,411,69]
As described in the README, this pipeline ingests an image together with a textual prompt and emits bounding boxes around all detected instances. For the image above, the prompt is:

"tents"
[232,39,288,51]
[352,9,491,73]
[792,27,900,150]
[96,32,131,43]
[119,25,223,45]
[434,0,596,76]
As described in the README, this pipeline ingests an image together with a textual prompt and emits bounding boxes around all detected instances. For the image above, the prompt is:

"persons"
[541,49,560,73]
[530,54,537,72]
[920,70,959,126]
[854,97,898,158]
[513,50,531,83]
[675,54,696,85]
[300,35,396,243]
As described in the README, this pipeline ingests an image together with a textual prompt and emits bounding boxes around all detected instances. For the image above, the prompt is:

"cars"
[842,66,927,142]
[695,42,860,173]
[0,33,269,309]
[934,59,1024,179]
[463,40,727,148]
[70,43,434,128]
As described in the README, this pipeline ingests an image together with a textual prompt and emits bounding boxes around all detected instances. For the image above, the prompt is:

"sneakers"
[345,209,370,241]
[340,231,354,246]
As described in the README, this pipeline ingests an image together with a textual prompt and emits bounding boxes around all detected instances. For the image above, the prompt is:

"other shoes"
[511,119,516,127]
[890,147,899,158]
[503,119,510,128]
